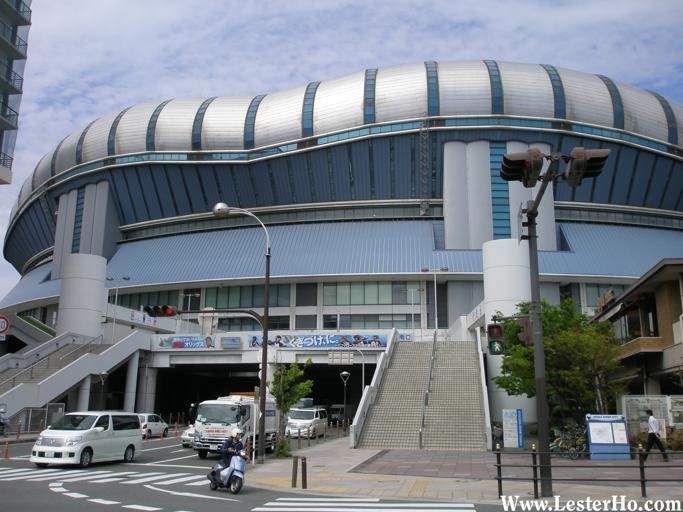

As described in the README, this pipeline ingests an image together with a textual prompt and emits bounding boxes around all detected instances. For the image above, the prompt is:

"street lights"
[104,276,132,347]
[399,287,425,342]
[417,266,449,336]
[338,371,353,438]
[212,199,273,464]
[179,292,201,332]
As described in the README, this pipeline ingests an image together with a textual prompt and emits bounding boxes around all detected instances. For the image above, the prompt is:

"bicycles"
[548,422,590,461]
[491,422,503,447]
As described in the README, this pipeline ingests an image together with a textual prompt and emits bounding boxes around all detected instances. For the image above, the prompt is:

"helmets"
[231,428,242,438]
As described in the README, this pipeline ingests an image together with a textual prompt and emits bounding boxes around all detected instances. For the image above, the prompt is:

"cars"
[180,424,195,448]
[136,411,170,440]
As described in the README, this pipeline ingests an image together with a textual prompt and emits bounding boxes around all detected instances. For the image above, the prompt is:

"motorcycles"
[207,444,256,496]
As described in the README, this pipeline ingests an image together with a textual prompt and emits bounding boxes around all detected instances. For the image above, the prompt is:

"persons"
[205,336,214,348]
[0,409,9,434]
[249,335,287,347]
[643,409,669,462]
[341,335,381,348]
[218,428,244,468]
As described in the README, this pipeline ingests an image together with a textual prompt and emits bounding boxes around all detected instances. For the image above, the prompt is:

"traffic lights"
[486,322,505,356]
[143,304,177,317]
[500,147,543,188]
[566,145,612,190]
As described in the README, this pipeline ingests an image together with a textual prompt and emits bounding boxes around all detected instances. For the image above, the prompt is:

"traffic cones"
[2,440,12,459]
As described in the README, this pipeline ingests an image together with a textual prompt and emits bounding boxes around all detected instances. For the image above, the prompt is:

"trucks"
[188,396,289,460]
[328,403,344,423]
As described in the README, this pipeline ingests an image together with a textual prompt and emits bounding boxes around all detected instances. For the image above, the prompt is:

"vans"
[26,410,144,470]
[286,407,328,439]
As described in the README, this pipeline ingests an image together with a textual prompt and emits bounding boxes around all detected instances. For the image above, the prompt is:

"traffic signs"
[0,314,10,333]
